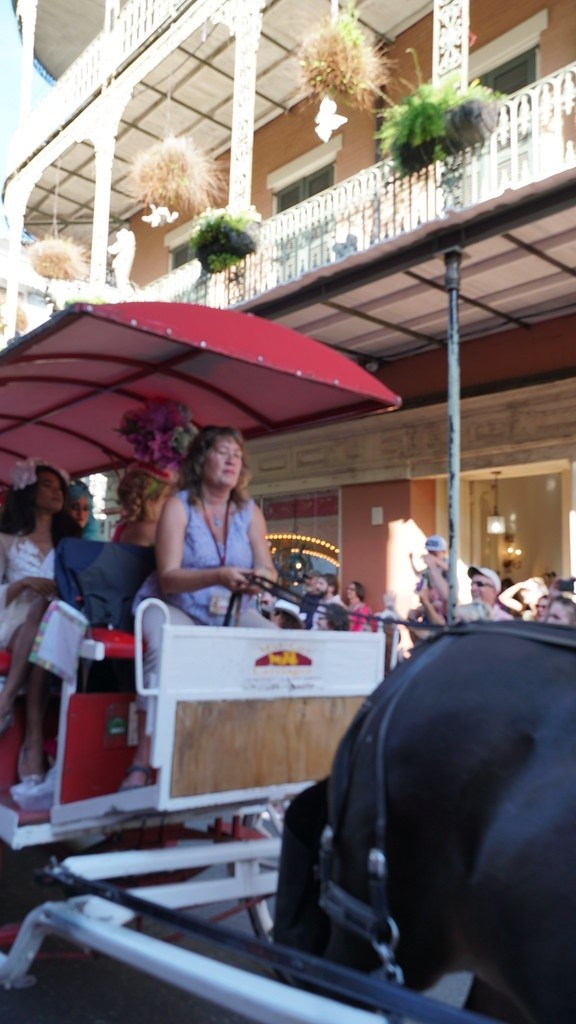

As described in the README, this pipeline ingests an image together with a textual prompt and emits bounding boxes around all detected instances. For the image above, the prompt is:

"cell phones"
[558,580,574,592]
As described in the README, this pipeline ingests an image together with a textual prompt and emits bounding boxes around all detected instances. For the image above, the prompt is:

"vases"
[196,230,256,272]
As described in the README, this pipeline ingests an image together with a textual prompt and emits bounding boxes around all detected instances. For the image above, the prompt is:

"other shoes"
[119,766,150,793]
[17,744,45,785]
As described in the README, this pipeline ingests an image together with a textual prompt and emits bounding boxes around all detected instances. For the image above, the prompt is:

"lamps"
[486,471,506,534]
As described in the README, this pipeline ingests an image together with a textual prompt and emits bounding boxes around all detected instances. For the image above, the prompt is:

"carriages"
[0,297,576,1024]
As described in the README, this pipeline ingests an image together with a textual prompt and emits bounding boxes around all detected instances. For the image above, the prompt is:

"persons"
[108,228,136,287]
[0,458,180,785]
[120,424,279,789]
[267,533,576,671]
[141,204,179,227]
[314,97,337,142]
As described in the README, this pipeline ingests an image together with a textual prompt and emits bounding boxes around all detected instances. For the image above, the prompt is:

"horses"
[276,618,576,1024]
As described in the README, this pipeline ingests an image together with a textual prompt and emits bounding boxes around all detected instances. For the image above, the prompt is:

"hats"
[262,599,307,621]
[468,566,500,593]
[304,569,322,579]
[425,536,447,552]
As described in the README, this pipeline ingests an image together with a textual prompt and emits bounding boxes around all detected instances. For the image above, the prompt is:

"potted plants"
[370,48,507,179]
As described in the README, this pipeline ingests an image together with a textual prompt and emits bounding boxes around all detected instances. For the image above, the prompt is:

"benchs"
[54,540,156,658]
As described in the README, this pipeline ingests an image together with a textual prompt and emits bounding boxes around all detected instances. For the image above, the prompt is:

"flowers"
[185,205,262,273]
[121,400,200,472]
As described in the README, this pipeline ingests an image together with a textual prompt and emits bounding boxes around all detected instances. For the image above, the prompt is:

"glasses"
[535,605,546,608]
[471,581,493,587]
[261,601,268,605]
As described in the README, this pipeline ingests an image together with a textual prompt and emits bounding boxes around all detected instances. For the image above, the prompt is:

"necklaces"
[205,499,226,527]
